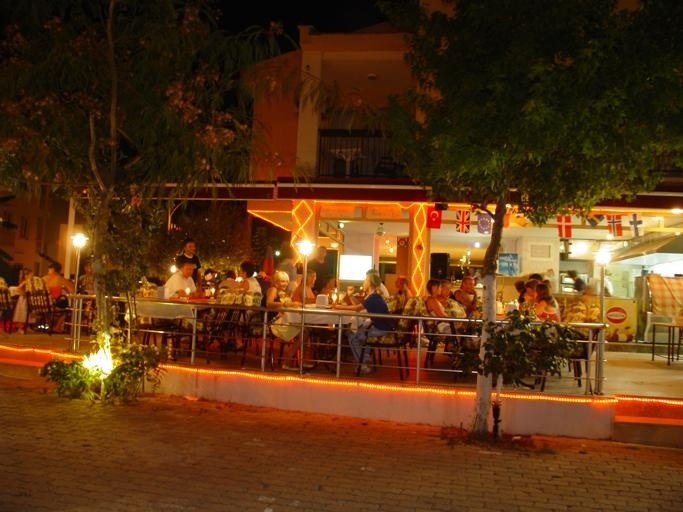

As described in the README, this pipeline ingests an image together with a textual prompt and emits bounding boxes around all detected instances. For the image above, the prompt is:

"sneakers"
[356,362,373,374]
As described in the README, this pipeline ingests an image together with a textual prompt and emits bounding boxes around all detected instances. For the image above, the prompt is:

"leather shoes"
[282,364,314,371]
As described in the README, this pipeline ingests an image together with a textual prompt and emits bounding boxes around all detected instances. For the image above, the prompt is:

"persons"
[515,272,560,318]
[424,275,478,352]
[23,268,33,280]
[1,278,12,321]
[163,240,412,375]
[593,270,614,297]
[42,262,73,309]
[567,271,587,295]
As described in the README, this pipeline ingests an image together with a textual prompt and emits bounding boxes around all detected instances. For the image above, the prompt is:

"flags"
[627,213,645,238]
[556,215,572,238]
[425,207,442,229]
[477,213,491,236]
[454,210,471,234]
[605,214,623,237]
[584,215,604,227]
